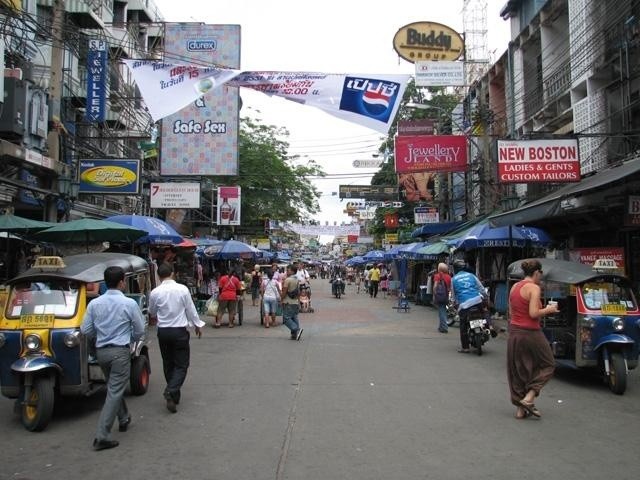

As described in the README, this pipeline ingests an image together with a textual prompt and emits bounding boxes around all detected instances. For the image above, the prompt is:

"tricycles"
[505,257,639,396]
[0,251,152,434]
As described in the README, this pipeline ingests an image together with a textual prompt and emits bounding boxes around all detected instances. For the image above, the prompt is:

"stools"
[195,299,207,315]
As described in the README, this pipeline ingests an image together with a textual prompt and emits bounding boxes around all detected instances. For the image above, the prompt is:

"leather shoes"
[164,392,178,413]
[93,438,119,451]
[119,413,131,431]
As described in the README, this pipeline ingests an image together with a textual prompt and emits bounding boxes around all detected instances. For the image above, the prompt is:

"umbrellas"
[0,206,289,262]
[344,218,553,262]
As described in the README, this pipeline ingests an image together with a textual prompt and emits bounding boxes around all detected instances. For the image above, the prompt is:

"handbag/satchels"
[287,287,298,299]
[434,273,449,305]
[368,278,371,286]
[274,287,281,302]
[259,277,261,285]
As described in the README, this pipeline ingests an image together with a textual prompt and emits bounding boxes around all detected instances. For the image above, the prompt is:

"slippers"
[213,321,280,328]
[516,408,533,418]
[490,328,497,338]
[458,348,470,353]
[520,400,541,417]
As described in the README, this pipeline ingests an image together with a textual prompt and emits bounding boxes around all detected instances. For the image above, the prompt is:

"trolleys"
[258,294,286,326]
[213,285,244,328]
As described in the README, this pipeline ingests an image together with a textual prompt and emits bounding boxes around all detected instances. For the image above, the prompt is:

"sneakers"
[295,328,303,340]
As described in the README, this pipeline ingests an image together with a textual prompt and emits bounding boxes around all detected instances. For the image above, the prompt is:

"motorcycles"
[470,321,491,352]
[440,289,464,326]
[331,274,345,299]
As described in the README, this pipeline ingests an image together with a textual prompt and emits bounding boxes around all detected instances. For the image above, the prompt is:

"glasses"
[538,271,543,274]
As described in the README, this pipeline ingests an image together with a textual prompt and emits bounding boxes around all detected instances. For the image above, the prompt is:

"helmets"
[453,258,466,275]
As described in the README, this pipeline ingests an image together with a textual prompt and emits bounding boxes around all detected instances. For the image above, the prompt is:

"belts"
[99,344,130,348]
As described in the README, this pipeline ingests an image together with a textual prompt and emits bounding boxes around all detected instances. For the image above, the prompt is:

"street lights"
[406,98,485,218]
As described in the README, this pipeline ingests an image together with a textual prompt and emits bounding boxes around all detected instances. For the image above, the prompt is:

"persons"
[507,259,560,419]
[213,262,312,340]
[433,262,452,333]
[316,263,393,298]
[149,262,206,413]
[80,265,145,448]
[450,258,497,353]
